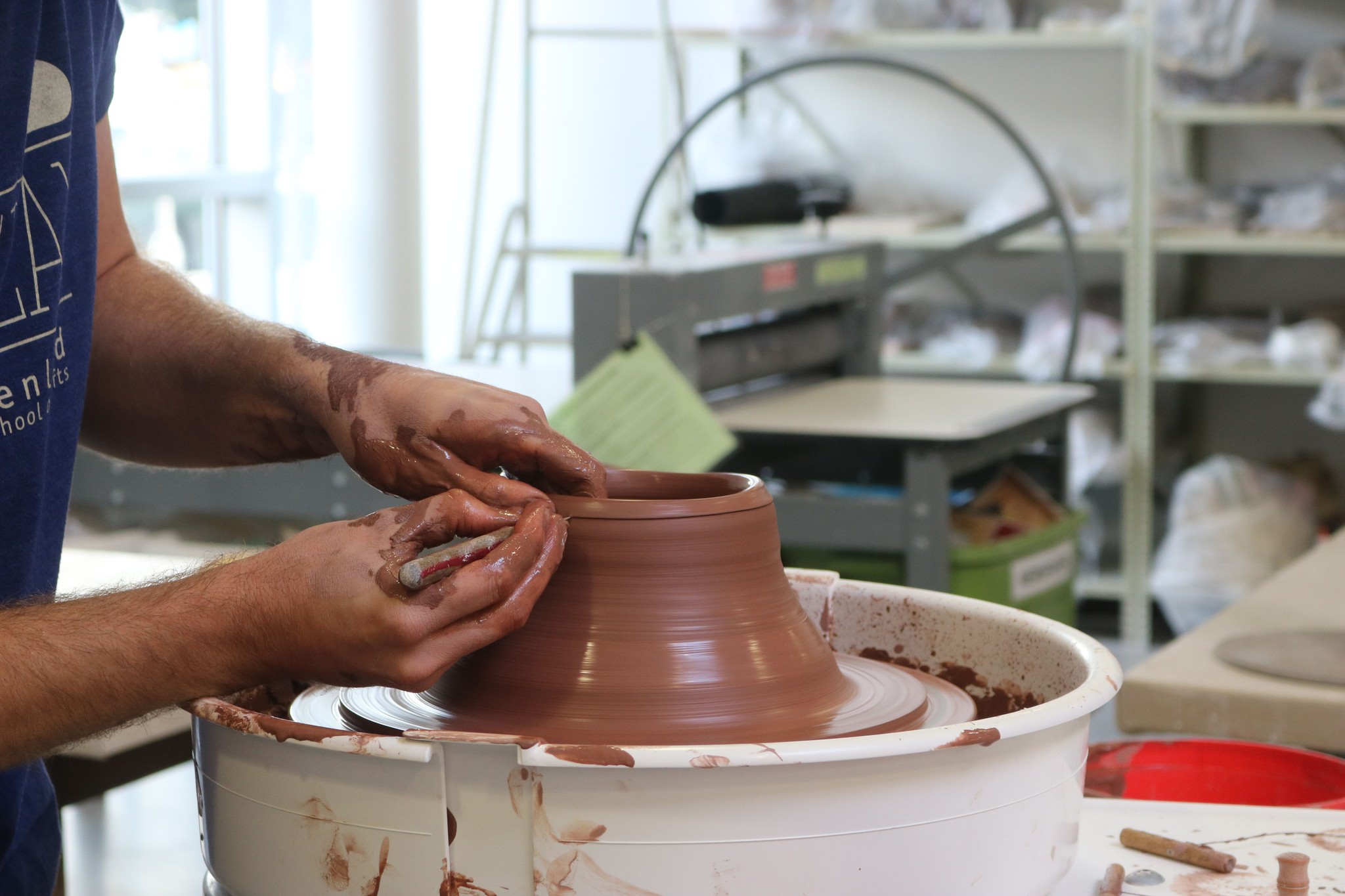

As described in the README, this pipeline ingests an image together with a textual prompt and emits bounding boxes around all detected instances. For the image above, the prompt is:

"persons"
[0,0,607,896]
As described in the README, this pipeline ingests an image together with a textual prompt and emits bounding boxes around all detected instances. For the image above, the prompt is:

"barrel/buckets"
[1084,735,1345,810]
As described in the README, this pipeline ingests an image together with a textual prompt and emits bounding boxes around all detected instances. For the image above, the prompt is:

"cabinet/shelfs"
[785,2,1345,648]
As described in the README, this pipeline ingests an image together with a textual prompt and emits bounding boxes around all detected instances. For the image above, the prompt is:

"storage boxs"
[787,510,1087,626]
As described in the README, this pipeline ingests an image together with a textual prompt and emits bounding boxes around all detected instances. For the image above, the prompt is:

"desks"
[1116,530,1345,763]
